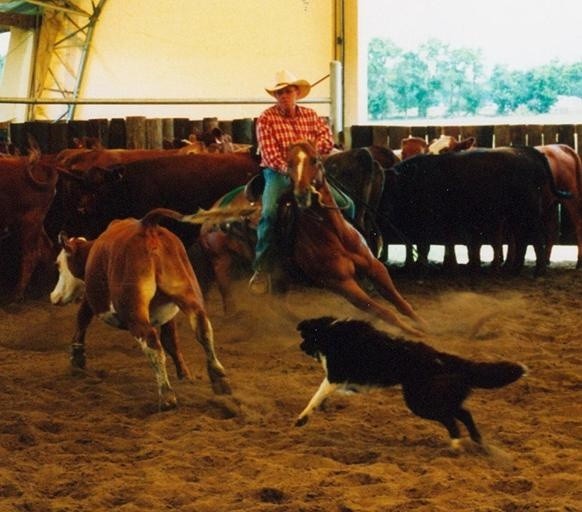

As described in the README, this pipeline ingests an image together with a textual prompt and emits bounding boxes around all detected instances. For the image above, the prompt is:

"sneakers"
[247,268,269,296]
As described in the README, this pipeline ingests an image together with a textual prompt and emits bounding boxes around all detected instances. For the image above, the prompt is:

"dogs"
[290,313,530,452]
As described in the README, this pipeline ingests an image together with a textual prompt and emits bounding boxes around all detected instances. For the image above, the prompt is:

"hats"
[264,69,311,100]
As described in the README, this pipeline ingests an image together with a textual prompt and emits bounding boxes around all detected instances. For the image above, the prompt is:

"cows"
[0,135,581,305]
[46,207,233,411]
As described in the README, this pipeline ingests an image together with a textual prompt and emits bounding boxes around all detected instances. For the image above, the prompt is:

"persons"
[174,127,236,152]
[0,130,99,155]
[246,69,377,297]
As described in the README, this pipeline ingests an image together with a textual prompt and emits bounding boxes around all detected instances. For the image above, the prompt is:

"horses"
[180,139,435,340]
